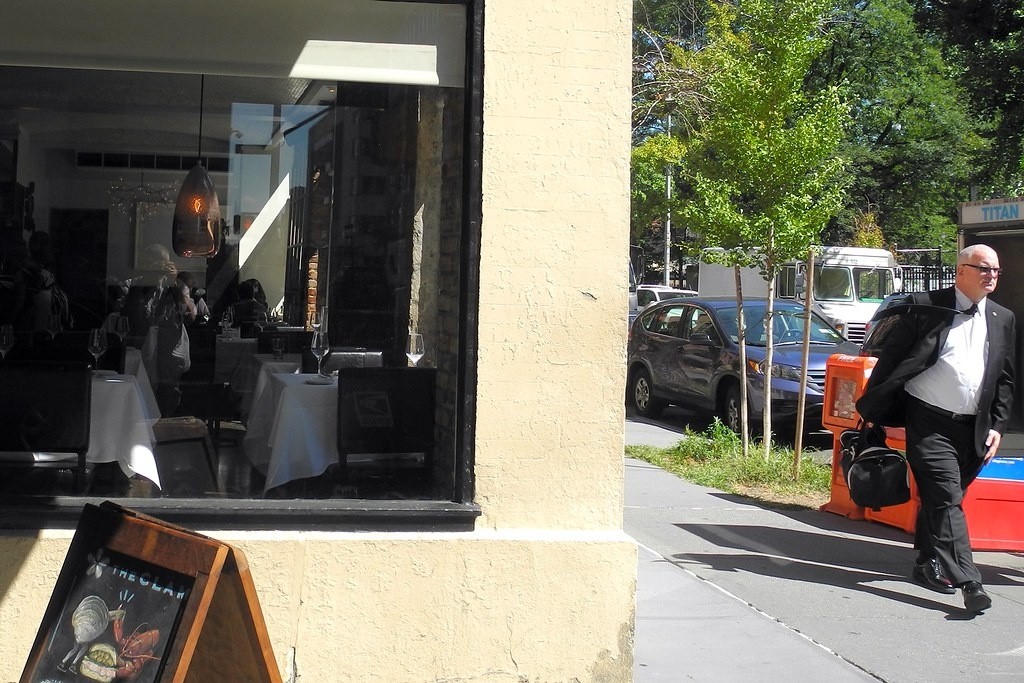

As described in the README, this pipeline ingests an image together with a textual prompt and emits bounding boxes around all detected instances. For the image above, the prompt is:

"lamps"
[104,169,179,228]
[172,72,221,259]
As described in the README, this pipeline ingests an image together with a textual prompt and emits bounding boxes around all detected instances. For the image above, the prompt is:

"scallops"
[69,595,110,644]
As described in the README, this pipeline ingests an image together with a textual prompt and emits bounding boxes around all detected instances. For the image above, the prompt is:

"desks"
[90,370,161,490]
[214,333,257,384]
[243,374,424,496]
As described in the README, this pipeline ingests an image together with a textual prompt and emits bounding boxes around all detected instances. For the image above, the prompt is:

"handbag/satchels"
[839,417,911,511]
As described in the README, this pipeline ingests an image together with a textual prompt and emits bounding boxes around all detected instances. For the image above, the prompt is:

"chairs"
[0,275,436,498]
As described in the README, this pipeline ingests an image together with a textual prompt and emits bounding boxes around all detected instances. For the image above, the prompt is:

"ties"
[872,303,978,322]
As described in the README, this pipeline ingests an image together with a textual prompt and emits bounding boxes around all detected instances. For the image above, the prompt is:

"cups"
[271,338,285,360]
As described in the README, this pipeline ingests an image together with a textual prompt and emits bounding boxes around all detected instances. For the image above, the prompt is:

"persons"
[228,279,270,330]
[13,229,69,333]
[138,302,192,416]
[855,244,1016,615]
[152,272,211,326]
[727,315,782,341]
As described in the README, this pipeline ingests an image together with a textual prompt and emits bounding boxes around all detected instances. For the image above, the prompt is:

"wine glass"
[220,311,234,341]
[309,330,331,380]
[404,333,426,368]
[87,328,107,380]
[114,316,129,342]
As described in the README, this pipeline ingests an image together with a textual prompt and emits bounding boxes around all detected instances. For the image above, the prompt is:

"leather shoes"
[962,581,991,618]
[913,556,955,594]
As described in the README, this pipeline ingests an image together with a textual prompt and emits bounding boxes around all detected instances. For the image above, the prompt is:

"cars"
[629,243,699,336]
[627,295,860,446]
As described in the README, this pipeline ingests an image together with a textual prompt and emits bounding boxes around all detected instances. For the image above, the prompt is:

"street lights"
[657,93,676,287]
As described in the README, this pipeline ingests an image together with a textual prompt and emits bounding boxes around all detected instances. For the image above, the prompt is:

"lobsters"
[110,609,161,681]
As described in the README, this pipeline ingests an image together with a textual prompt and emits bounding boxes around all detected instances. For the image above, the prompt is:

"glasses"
[962,263,1004,275]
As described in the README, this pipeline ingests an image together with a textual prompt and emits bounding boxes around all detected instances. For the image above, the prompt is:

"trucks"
[699,245,903,351]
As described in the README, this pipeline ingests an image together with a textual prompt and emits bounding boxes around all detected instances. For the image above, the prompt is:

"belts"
[907,393,976,422]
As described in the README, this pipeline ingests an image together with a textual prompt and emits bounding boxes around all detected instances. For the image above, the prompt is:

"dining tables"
[233,352,301,424]
[124,348,160,426]
